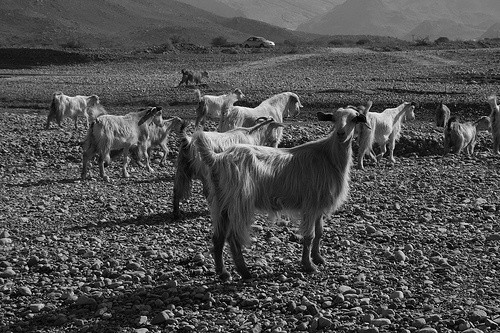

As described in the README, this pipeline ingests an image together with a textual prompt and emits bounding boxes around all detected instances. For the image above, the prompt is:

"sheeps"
[44,69,500,284]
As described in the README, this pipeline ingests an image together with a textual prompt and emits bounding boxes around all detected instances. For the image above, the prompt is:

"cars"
[241,36,275,48]
